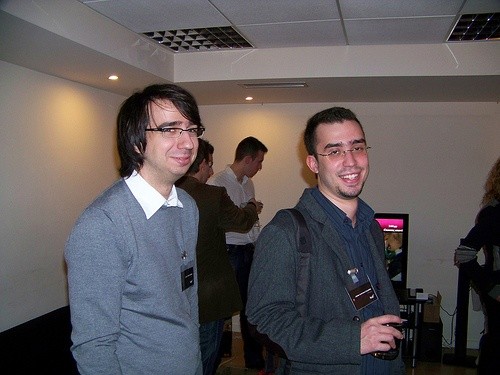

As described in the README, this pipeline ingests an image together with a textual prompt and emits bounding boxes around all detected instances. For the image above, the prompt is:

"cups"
[371,323,402,360]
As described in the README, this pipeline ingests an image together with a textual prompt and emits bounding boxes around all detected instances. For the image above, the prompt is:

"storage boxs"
[424,291,442,323]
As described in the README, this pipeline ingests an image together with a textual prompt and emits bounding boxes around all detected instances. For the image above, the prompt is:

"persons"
[453,154,500,375]
[385,232,404,276]
[174,138,264,375]
[205,136,269,374]
[244,106,407,375]
[63,82,208,375]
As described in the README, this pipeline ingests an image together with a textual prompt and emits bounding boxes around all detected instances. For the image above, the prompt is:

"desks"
[396,295,427,368]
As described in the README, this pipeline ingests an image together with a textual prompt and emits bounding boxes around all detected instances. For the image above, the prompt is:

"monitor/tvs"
[373,213,409,289]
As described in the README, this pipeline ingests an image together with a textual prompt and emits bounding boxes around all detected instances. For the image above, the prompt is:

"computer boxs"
[415,312,443,363]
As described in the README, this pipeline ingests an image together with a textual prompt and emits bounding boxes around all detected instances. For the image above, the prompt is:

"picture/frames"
[374,214,408,288]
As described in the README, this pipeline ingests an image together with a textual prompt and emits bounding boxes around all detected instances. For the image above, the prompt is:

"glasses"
[314,145,372,161]
[145,122,207,139]
